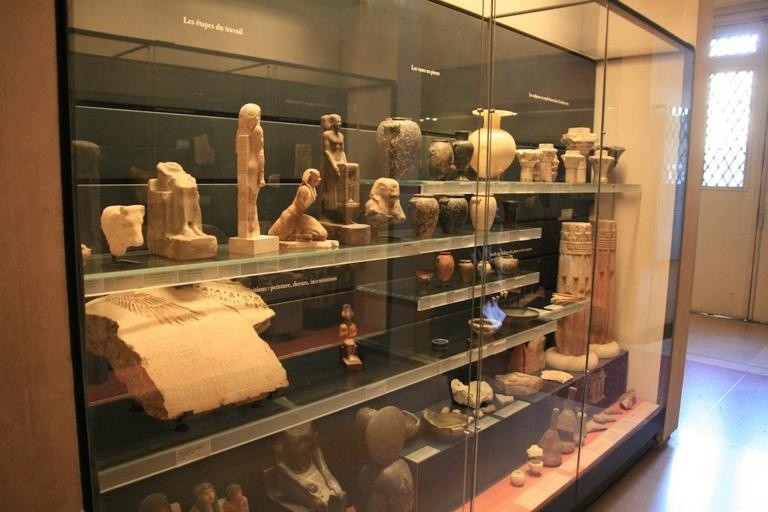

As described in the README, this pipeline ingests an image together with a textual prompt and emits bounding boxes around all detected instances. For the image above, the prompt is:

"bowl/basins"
[469,317,501,337]
[402,403,470,442]
[496,305,539,326]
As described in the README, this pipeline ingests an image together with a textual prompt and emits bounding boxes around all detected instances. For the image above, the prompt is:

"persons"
[234,102,267,237]
[320,112,356,206]
[191,482,221,512]
[337,297,363,374]
[268,167,328,241]
[218,483,250,512]
[273,420,347,512]
